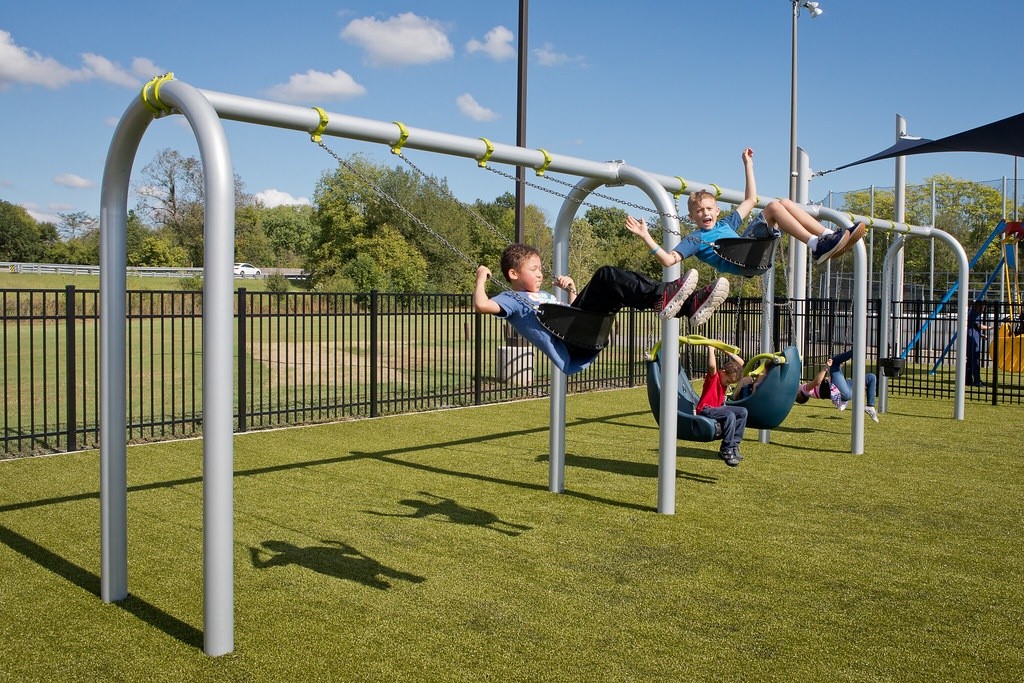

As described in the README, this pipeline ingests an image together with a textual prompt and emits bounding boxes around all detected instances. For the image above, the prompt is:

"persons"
[472,242,729,375]
[728,359,776,402]
[624,146,865,277]
[796,359,880,423]
[697,346,748,467]
[1003,315,1015,336]
[966,301,1002,386]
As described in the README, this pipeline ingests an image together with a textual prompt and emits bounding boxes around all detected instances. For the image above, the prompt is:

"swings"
[817,223,875,400]
[878,232,910,379]
[725,207,800,434]
[480,160,781,273]
[310,134,614,364]
[640,194,746,444]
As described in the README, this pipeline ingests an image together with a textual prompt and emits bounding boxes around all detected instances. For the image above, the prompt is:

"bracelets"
[651,245,660,255]
[823,366,829,372]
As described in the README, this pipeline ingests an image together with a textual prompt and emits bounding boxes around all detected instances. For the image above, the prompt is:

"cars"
[234,262,261,276]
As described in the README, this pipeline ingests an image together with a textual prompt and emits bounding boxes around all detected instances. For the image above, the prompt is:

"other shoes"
[975,381,986,387]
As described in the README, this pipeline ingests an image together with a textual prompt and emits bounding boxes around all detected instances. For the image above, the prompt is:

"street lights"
[789,0,823,299]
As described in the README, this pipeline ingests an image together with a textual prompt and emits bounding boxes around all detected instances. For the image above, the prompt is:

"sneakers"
[811,229,850,265]
[686,277,730,325]
[733,447,744,461]
[730,385,735,391]
[654,268,698,321]
[865,405,879,423]
[718,447,739,466]
[829,221,865,258]
